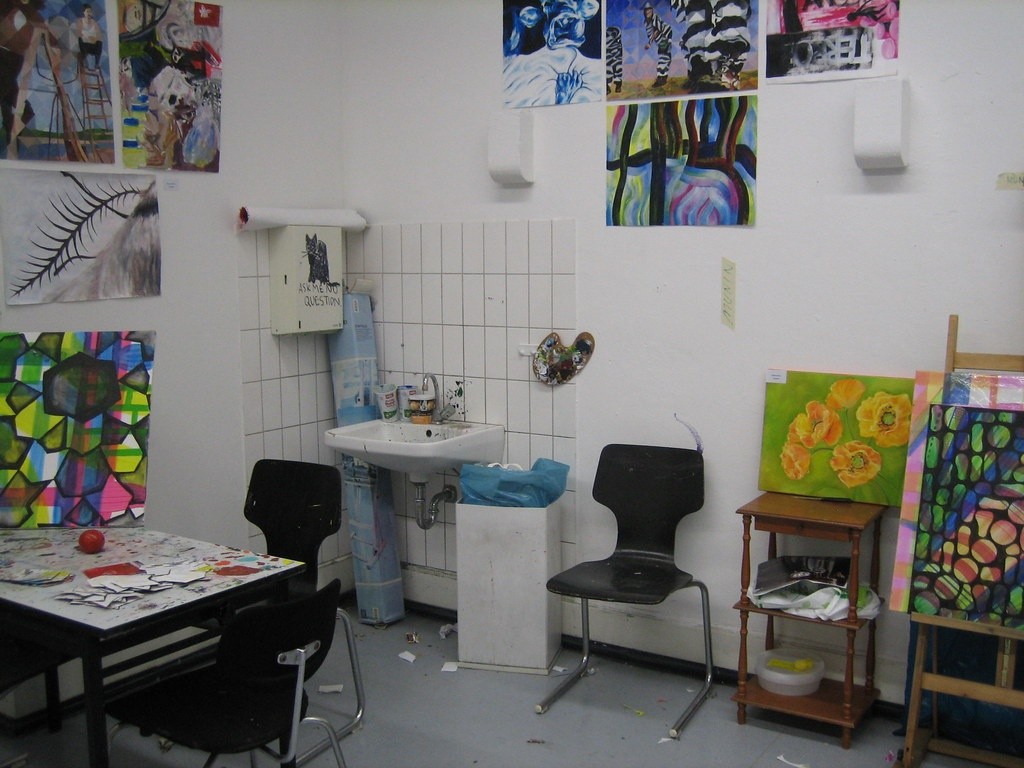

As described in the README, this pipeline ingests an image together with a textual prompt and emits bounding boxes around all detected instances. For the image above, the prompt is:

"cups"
[374,384,399,423]
[409,395,435,424]
[397,386,418,422]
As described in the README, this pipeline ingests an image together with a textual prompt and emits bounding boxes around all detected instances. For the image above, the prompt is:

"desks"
[0,526,306,768]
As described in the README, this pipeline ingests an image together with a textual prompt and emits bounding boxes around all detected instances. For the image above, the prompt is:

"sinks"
[325,419,506,473]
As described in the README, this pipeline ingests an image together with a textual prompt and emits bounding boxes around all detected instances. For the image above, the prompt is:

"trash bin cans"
[455,457,571,676]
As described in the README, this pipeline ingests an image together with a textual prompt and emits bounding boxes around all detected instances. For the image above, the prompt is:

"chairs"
[188,458,366,766]
[108,574,342,768]
[534,443,716,739]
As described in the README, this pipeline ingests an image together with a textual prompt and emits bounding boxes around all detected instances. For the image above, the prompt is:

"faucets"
[422,373,455,425]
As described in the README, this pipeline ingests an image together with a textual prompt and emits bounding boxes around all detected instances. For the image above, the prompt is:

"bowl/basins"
[754,648,825,696]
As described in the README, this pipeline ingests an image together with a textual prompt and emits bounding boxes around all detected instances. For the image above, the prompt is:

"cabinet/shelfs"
[733,488,886,749]
